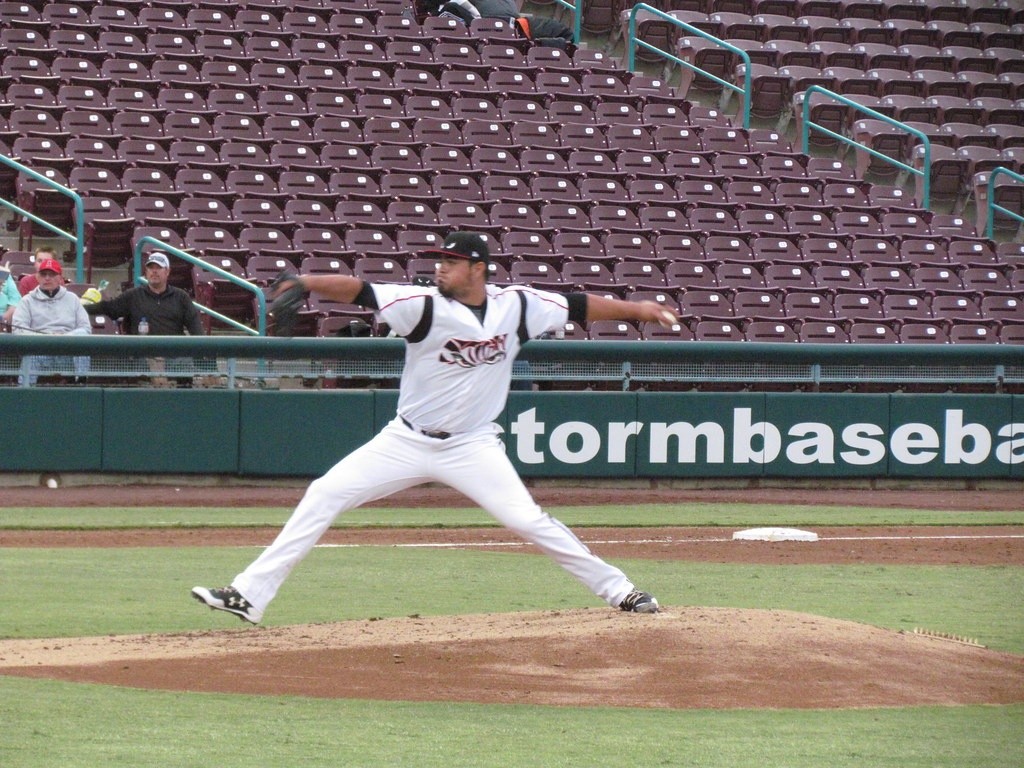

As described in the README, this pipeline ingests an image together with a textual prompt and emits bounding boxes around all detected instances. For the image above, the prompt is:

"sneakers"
[191,585,263,626]
[619,588,659,612]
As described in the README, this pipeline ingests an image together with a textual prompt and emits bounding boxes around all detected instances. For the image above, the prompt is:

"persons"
[0,245,93,389]
[82,251,221,387]
[191,230,677,627]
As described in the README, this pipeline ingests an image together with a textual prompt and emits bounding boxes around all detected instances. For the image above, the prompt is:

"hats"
[38,258,62,274]
[145,252,169,269]
[416,232,489,263]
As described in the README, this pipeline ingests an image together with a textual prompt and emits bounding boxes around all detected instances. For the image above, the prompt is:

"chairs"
[0,1,1023,393]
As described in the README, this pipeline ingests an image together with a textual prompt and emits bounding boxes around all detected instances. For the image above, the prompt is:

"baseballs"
[660,310,680,332]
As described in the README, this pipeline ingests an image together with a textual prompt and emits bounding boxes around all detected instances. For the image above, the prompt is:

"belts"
[401,416,451,439]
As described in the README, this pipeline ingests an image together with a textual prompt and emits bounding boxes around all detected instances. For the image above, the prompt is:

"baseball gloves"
[262,273,307,336]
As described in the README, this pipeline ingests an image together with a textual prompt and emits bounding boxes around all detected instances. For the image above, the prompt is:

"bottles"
[0,316,8,332]
[138,317,149,335]
